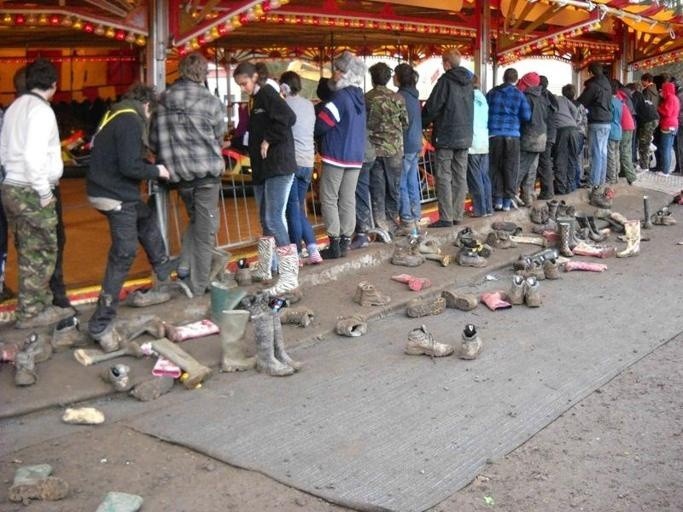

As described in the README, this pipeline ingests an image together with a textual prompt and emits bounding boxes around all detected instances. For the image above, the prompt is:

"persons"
[234,48,422,298]
[86,83,180,334]
[421,48,681,230]
[151,52,229,297]
[1,59,78,329]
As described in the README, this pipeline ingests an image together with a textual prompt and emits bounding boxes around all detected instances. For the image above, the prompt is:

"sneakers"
[441,290,478,311]
[353,282,391,306]
[337,315,368,337]
[406,297,447,317]
[461,324,483,359]
[405,325,454,356]
[467,165,683,311]
[319,220,491,268]
[16,306,95,385]
[63,408,104,424]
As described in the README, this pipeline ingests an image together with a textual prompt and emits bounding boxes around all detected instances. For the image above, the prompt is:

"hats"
[327,52,366,91]
[518,72,540,92]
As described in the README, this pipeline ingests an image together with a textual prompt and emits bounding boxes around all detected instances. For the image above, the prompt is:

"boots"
[77,237,323,401]
[392,274,432,291]
[96,491,142,512]
[9,464,68,502]
